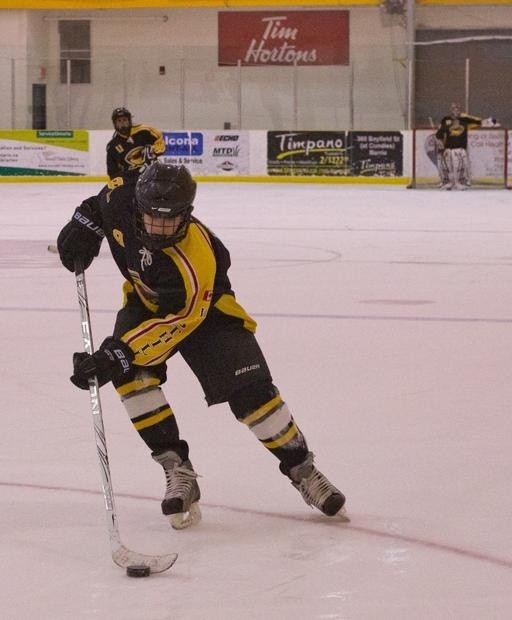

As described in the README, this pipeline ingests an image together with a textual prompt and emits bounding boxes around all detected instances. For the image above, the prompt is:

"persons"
[106,108,166,181]
[56,163,346,517]
[435,102,497,191]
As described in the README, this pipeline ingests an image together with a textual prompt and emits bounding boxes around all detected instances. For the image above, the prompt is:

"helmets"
[133,161,196,251]
[112,107,132,134]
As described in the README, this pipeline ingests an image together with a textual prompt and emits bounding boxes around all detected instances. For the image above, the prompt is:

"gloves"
[70,336,135,390]
[57,201,106,272]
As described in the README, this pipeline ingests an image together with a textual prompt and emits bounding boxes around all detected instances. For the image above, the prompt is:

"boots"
[151,440,200,515]
[280,451,345,517]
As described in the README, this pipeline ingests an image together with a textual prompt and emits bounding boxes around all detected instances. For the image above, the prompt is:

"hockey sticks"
[71,248,177,573]
[46,245,60,254]
